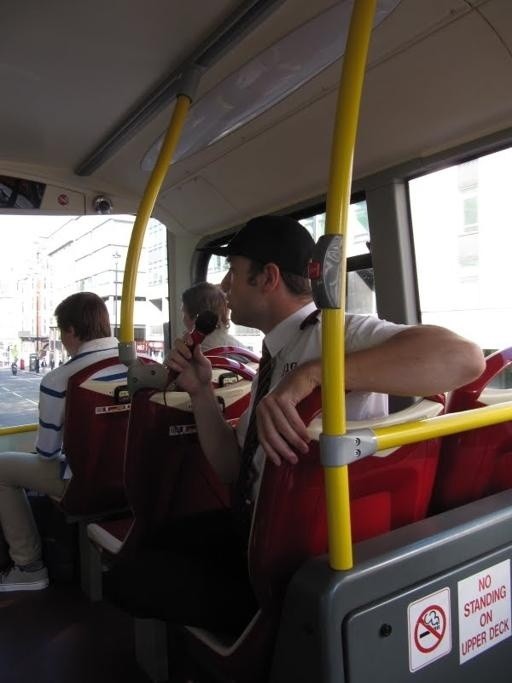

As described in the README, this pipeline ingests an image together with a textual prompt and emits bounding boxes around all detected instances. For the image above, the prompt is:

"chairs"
[36,346,512,683]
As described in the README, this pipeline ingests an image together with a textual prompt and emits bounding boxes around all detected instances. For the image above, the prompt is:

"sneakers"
[1,565,51,593]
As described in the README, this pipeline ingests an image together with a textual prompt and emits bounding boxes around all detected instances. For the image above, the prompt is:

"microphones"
[166,310,218,387]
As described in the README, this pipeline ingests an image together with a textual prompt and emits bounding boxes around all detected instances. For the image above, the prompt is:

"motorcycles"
[13,366,17,375]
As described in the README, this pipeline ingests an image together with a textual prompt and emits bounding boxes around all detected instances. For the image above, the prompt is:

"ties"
[236,341,274,504]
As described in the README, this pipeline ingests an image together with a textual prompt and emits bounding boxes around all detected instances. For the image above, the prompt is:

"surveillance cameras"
[96,196,113,215]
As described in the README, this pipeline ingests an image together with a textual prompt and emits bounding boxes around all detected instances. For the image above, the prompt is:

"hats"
[195,215,316,277]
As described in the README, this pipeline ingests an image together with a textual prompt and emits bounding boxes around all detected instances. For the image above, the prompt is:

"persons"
[49,357,55,370]
[177,281,253,365]
[42,357,47,374]
[11,361,18,376]
[0,291,129,595]
[103,215,488,630]
[59,359,64,365]
